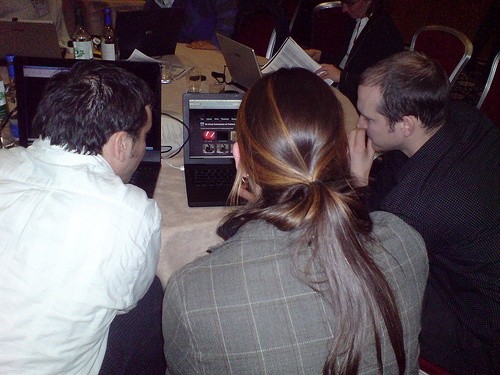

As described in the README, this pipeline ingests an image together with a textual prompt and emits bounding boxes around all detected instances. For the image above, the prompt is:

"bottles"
[5,55,19,141]
[72,7,94,59]
[100,7,118,61]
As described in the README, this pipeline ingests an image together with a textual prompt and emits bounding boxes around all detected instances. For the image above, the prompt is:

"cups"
[158,60,172,84]
[208,82,225,94]
[186,71,201,92]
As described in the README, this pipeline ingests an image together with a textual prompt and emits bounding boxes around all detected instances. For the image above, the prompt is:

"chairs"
[232,0,500,130]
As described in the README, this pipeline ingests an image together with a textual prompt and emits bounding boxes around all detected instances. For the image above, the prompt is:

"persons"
[0,61,162,375]
[160,67,430,375]
[346,53,500,375]
[303,0,405,116]
[144,0,239,48]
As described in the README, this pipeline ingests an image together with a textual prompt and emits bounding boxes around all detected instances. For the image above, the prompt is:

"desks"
[150,43,379,291]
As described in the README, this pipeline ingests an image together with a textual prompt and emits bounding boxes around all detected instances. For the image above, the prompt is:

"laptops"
[181,92,248,207]
[13,56,162,199]
[215,33,264,92]
[0,20,66,65]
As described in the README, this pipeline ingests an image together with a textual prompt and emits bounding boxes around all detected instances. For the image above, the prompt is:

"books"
[258,37,334,86]
